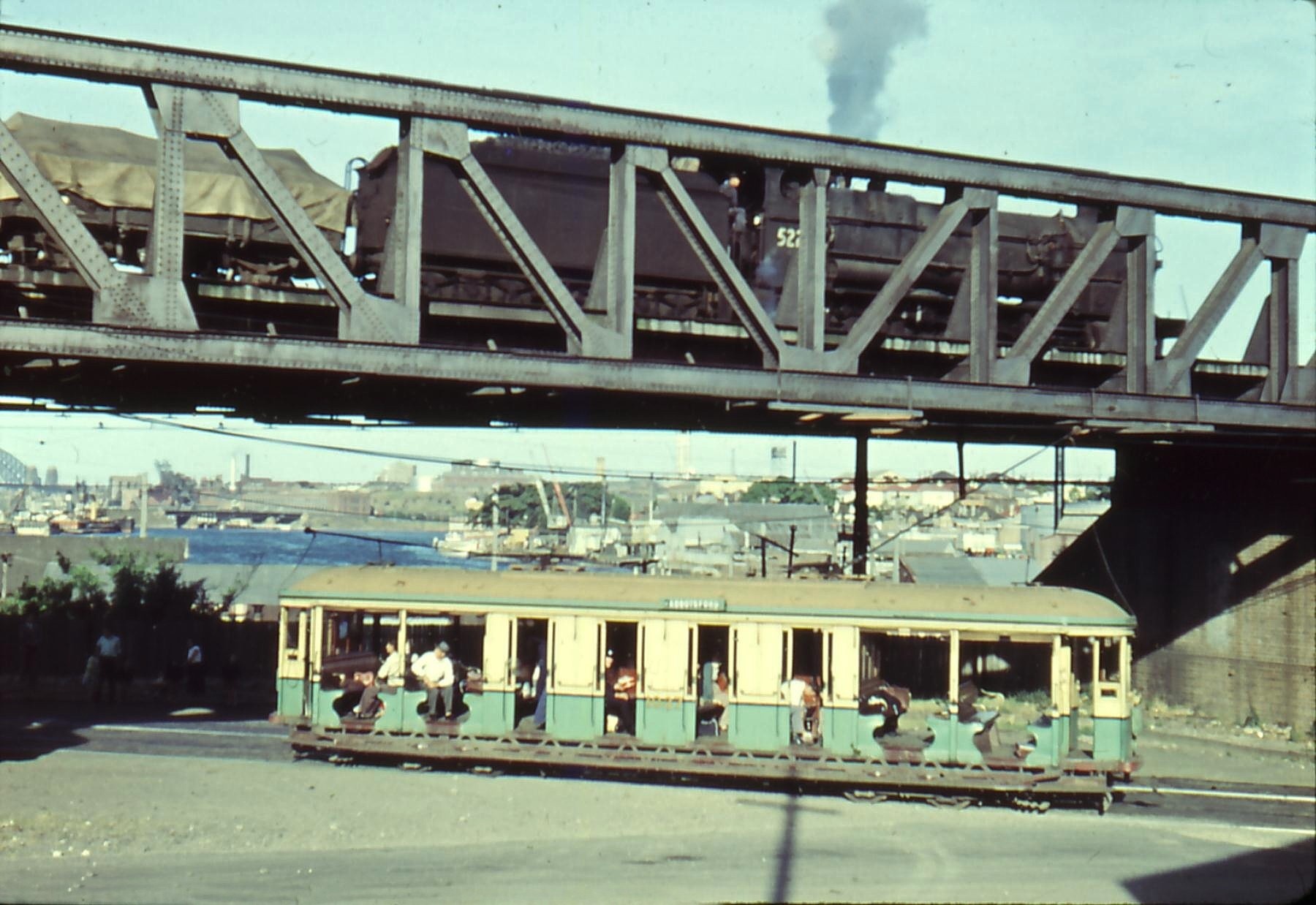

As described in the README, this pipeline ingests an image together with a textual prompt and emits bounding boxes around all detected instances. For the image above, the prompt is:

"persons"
[351,639,400,720]
[178,637,206,696]
[92,624,122,700]
[411,640,455,723]
[531,637,546,725]
[779,678,819,746]
[598,648,638,736]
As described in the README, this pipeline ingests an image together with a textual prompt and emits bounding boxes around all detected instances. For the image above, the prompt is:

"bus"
[262,565,1151,811]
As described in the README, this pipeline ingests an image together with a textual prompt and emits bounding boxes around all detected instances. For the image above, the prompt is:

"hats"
[435,641,451,655]
[607,649,615,658]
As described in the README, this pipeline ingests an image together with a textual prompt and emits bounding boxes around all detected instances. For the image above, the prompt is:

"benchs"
[607,668,637,731]
[1023,710,1053,763]
[958,681,998,752]
[322,651,381,718]
[859,676,911,739]
[794,675,823,735]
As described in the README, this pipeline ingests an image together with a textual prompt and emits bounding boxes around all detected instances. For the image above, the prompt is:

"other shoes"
[446,715,456,723]
[425,715,434,723]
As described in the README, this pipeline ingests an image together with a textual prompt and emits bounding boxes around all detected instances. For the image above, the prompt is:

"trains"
[1,94,1166,385]
